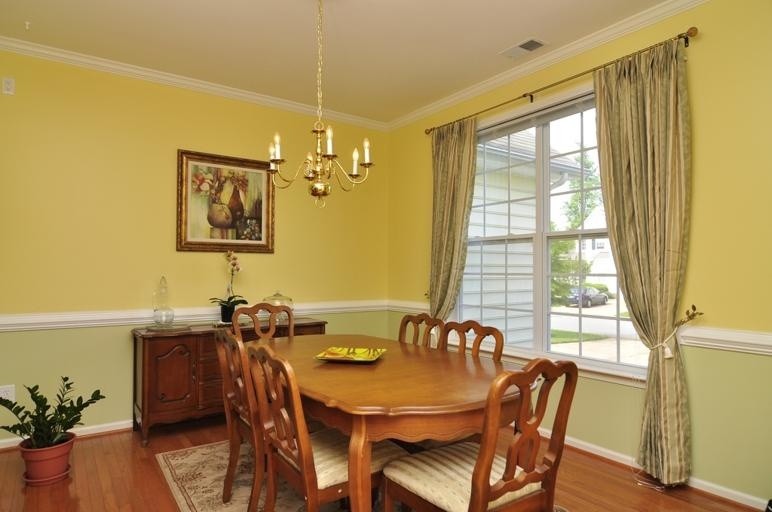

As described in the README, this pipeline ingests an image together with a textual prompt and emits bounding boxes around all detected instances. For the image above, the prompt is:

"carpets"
[154,436,416,511]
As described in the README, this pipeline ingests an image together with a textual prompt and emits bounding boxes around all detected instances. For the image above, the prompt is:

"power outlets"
[2,384,17,405]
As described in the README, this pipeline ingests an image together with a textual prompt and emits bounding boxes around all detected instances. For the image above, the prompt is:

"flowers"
[208,249,249,308]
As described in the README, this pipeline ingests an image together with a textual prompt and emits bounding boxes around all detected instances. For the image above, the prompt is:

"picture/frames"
[175,149,275,254]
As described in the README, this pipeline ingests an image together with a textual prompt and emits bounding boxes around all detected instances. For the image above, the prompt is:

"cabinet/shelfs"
[131,317,327,447]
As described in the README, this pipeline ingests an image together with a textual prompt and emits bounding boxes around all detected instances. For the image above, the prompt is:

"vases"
[218,305,235,324]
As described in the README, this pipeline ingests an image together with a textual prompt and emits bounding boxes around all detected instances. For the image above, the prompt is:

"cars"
[564,286,609,307]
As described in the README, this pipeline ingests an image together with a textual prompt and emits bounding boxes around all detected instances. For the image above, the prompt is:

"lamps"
[266,1,375,208]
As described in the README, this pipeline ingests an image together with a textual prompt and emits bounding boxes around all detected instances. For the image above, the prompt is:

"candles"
[268,142,276,168]
[323,124,334,155]
[351,146,359,171]
[274,131,281,159]
[304,151,315,175]
[363,138,370,165]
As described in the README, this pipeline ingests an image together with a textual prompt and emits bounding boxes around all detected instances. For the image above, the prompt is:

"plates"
[311,344,388,362]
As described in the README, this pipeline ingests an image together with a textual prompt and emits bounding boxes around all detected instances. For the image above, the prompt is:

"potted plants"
[1,376,106,484]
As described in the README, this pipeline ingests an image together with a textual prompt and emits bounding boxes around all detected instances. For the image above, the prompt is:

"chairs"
[381,358,579,511]
[231,301,295,402]
[398,313,445,353]
[212,329,315,511]
[404,320,504,450]
[247,344,410,511]
